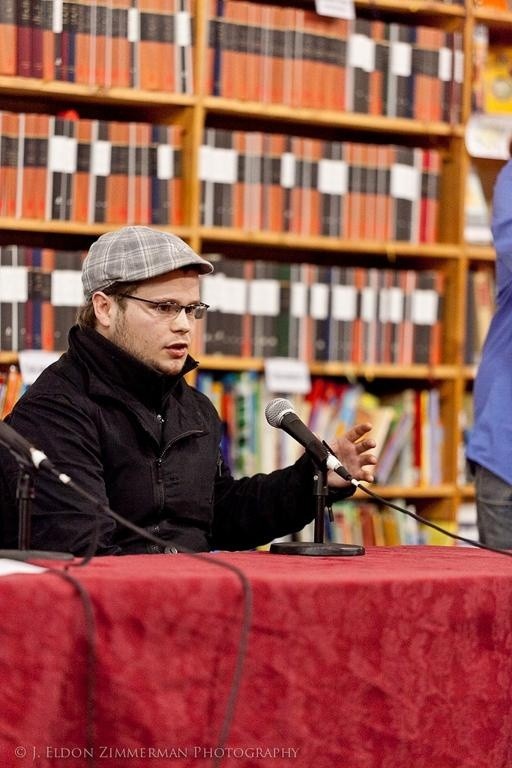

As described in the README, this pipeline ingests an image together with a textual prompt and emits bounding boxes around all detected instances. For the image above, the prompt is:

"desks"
[0,546,512,768]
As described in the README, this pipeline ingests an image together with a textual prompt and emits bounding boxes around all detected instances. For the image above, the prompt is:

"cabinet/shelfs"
[0,0,511,553]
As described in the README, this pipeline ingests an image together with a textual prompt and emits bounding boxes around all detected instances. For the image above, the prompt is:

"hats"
[84,227,217,301]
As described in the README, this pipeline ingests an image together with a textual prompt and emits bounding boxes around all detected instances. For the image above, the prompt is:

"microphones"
[264,397,351,481]
[0,419,55,476]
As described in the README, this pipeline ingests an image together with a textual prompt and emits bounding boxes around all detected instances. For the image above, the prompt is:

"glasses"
[115,289,208,323]
